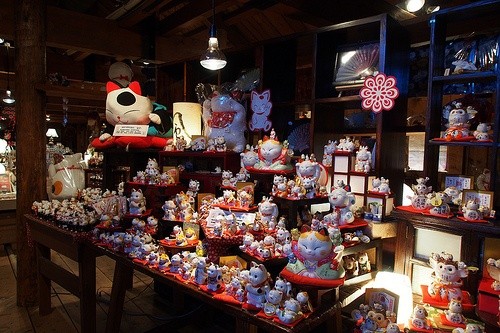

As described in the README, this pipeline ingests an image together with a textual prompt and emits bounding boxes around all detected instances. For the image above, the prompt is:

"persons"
[2,129,16,147]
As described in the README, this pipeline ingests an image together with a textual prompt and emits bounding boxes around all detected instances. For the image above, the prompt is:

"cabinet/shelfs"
[309,13,410,183]
[423,0,500,204]
[181,37,297,131]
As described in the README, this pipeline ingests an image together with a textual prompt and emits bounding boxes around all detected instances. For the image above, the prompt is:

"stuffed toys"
[99,77,173,141]
[47,152,85,202]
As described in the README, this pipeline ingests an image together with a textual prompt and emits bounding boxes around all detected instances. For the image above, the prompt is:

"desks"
[91,224,366,333]
[23,203,124,333]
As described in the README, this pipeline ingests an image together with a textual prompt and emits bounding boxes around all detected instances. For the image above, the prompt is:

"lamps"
[200,0,228,71]
[2,41,15,104]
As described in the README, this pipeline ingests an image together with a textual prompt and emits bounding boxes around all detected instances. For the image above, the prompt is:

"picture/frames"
[330,154,369,197]
[364,195,387,218]
[461,189,495,211]
[440,173,475,193]
